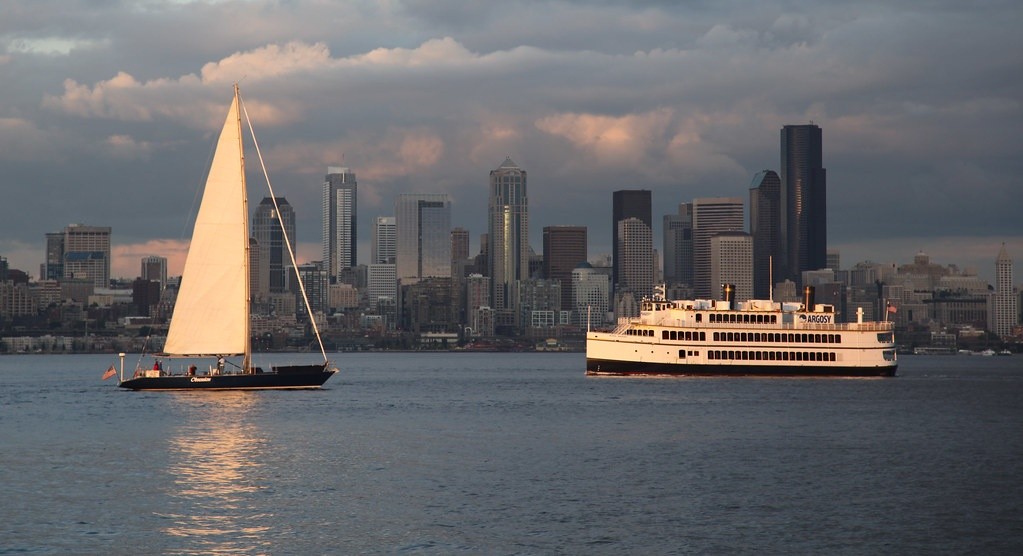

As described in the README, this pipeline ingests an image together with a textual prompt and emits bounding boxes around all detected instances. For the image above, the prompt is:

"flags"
[102,364,118,380]
[887,304,896,313]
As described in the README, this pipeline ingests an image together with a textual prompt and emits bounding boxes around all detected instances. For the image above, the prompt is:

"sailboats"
[110,86,340,391]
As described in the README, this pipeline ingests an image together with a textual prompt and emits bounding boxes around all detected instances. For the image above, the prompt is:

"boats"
[585,282,899,377]
[998,350,1012,355]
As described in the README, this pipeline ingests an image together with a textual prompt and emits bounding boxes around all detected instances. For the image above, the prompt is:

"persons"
[153,358,167,376]
[217,354,225,375]
[189,364,197,377]
